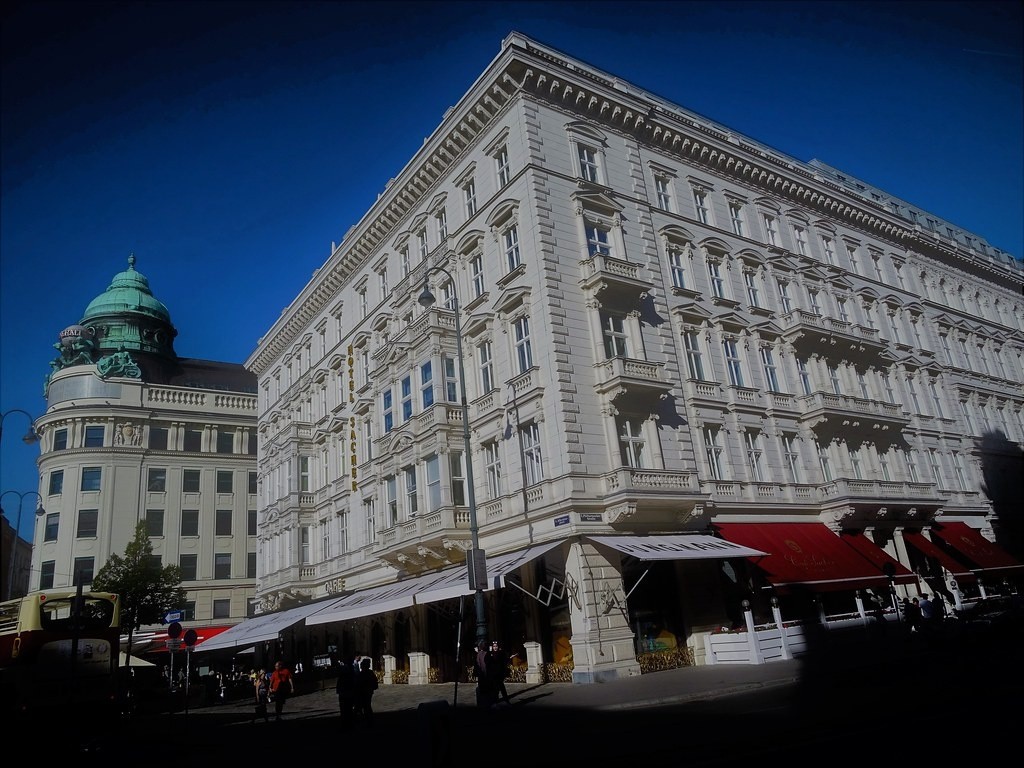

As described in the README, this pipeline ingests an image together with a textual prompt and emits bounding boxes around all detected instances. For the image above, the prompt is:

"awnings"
[712,523,919,596]
[585,536,772,561]
[193,593,350,651]
[305,539,568,627]
[904,522,1023,583]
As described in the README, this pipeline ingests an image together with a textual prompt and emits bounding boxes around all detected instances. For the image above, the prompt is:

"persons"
[474,639,511,706]
[903,587,956,627]
[163,665,184,691]
[249,661,294,724]
[1003,581,1011,596]
[336,655,379,718]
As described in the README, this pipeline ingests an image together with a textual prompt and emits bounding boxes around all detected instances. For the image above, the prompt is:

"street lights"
[0,408,39,445]
[0,490,46,600]
[416,267,491,648]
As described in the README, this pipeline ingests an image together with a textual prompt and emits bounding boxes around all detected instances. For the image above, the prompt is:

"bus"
[0,586,119,742]
